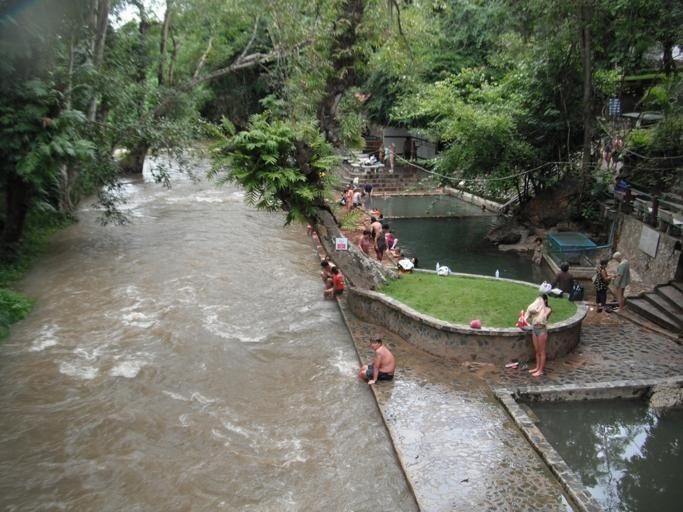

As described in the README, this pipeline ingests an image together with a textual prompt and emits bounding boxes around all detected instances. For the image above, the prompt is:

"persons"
[589,133,631,191]
[320,254,344,299]
[356,336,395,385]
[612,252,630,311]
[593,259,616,311]
[479,204,486,211]
[409,257,418,265]
[374,224,388,261]
[340,144,398,252]
[528,296,552,377]
[549,262,574,293]
